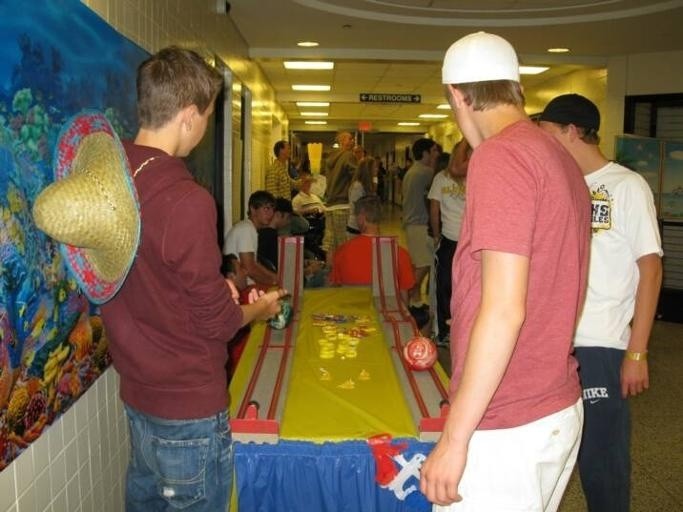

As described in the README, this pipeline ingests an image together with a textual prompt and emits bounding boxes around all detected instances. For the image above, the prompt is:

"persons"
[531,94,666,512]
[402,136,471,346]
[328,195,430,341]
[417,29,593,512]
[222,140,325,364]
[97,43,289,512]
[324,131,389,255]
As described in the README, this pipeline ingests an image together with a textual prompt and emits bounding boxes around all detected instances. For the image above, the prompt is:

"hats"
[440,31,521,84]
[540,93,600,129]
[32,107,142,305]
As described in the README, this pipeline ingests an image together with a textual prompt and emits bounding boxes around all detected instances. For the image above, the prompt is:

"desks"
[229,286,451,512]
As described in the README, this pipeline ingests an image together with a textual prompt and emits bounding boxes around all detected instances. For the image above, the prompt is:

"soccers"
[404,337,438,370]
[267,300,293,330]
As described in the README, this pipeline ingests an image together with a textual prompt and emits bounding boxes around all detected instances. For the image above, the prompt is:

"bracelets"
[625,346,649,364]
